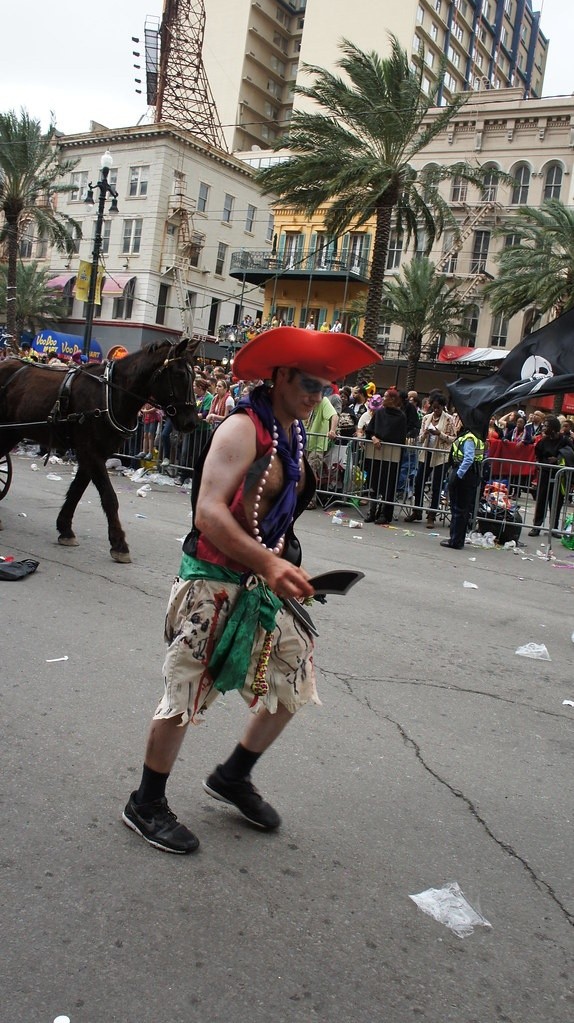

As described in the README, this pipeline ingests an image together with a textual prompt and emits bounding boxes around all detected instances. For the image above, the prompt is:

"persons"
[121,326,384,854]
[302,377,574,539]
[427,421,484,550]
[115,364,274,488]
[238,314,343,343]
[0,333,89,462]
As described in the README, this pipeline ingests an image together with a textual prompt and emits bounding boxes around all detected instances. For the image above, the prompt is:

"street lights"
[81,144,118,358]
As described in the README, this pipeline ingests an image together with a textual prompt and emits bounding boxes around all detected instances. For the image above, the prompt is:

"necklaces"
[252,416,303,555]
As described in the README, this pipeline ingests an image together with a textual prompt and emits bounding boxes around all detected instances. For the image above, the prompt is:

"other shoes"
[359,497,367,505]
[305,500,317,510]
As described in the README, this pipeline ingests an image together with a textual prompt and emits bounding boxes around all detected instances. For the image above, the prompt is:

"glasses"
[291,369,334,399]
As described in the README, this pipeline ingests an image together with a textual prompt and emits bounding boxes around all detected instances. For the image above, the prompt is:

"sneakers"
[174,479,183,485]
[123,789,201,854]
[551,532,562,538]
[405,511,422,522]
[426,516,435,528]
[161,458,170,466]
[202,765,282,830]
[528,528,540,535]
[374,513,392,524]
[364,512,377,522]
[135,451,146,458]
[144,454,153,461]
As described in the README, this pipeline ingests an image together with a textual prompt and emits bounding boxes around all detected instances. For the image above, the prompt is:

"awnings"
[41,274,136,297]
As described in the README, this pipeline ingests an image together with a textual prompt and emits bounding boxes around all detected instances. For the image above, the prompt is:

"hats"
[231,326,382,381]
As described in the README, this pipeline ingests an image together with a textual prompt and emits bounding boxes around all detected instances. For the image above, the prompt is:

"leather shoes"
[441,539,463,549]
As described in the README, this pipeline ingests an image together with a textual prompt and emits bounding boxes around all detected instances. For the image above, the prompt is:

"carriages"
[0,333,199,564]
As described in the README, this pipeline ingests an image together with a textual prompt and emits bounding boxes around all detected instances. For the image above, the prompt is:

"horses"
[0,338,204,564]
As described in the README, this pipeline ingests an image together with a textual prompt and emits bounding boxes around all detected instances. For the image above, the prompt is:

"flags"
[446,306,574,442]
